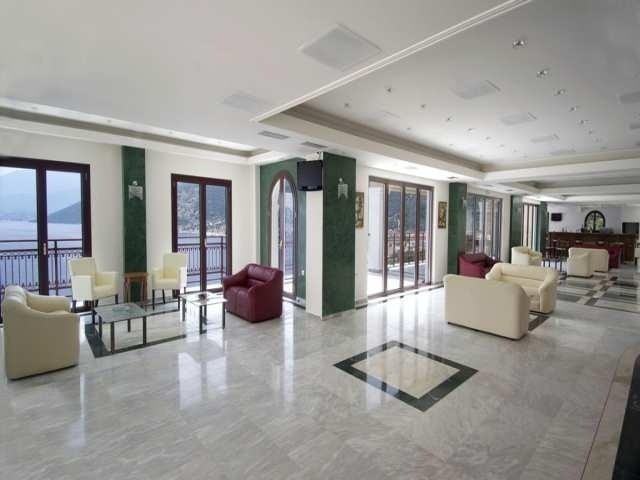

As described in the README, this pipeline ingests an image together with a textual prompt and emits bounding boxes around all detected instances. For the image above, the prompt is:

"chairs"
[566,251,594,278]
[66,257,120,325]
[0,284,80,380]
[222,263,284,323]
[151,253,187,311]
[511,246,543,266]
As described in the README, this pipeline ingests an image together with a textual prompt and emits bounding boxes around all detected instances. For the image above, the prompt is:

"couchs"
[568,246,610,273]
[458,252,496,278]
[485,262,557,314]
[581,242,624,268]
[442,274,530,341]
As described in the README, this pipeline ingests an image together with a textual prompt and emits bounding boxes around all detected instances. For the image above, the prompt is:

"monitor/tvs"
[297,161,323,191]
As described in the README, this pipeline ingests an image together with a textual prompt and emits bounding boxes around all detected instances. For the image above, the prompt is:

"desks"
[123,272,150,302]
